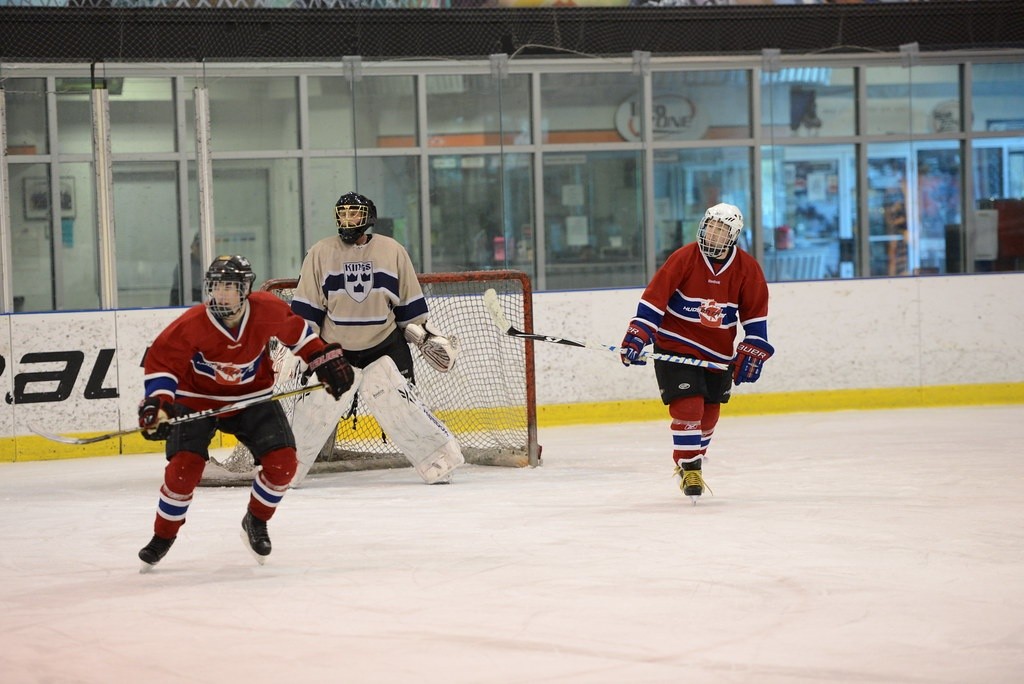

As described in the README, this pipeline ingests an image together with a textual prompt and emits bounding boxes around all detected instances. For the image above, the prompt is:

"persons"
[279,192,466,489]
[469,202,502,262]
[622,202,774,495]
[138,254,354,575]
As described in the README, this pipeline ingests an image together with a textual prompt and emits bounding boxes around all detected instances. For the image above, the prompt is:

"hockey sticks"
[26,381,332,446]
[484,288,731,372]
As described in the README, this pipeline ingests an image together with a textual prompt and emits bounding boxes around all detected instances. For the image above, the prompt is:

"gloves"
[405,320,457,374]
[727,336,775,385]
[137,389,175,441]
[307,343,355,401]
[619,320,655,367]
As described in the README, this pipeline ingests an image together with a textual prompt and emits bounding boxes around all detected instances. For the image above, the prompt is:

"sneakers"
[241,510,272,556]
[678,455,704,497]
[138,533,177,565]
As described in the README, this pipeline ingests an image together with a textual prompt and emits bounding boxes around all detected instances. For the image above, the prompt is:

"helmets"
[335,191,378,244]
[696,203,744,258]
[203,254,257,318]
